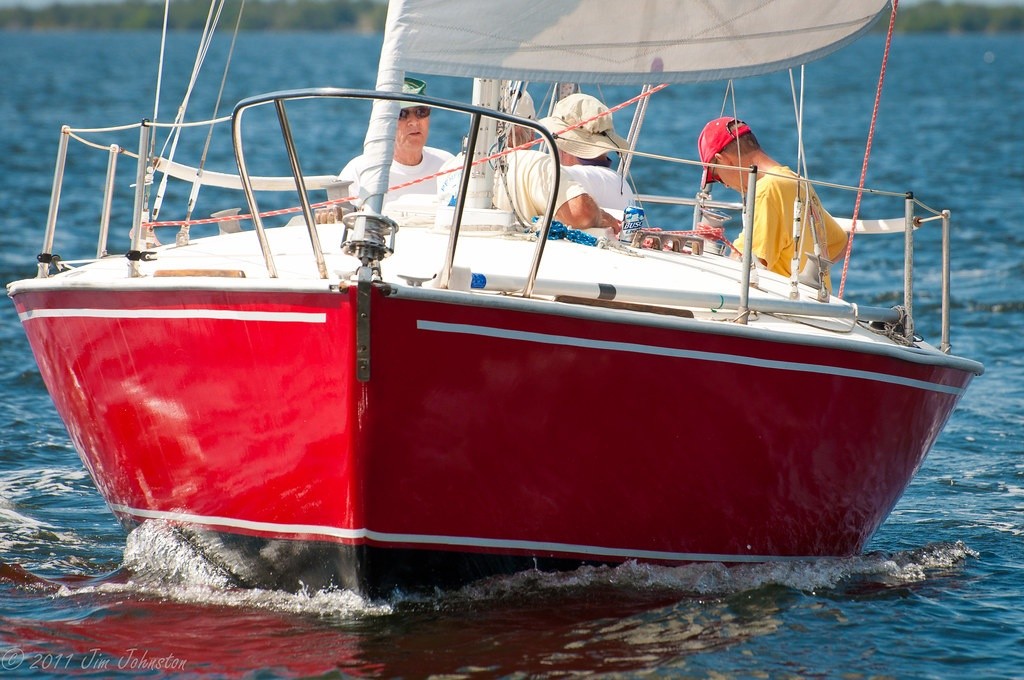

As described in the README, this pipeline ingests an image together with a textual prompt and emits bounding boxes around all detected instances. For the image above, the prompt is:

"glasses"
[707,159,725,185]
[396,106,431,121]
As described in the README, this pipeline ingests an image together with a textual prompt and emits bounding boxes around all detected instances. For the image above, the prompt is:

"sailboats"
[7,0,992,598]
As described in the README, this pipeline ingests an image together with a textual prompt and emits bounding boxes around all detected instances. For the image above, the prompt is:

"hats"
[394,75,428,108]
[533,94,632,161]
[498,89,537,123]
[696,119,751,188]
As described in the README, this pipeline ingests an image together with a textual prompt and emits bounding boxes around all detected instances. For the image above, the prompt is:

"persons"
[700,116,850,295]
[341,78,456,208]
[546,93,636,242]
[438,87,625,238]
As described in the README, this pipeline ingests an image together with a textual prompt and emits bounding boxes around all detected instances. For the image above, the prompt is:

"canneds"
[617,206,646,245]
